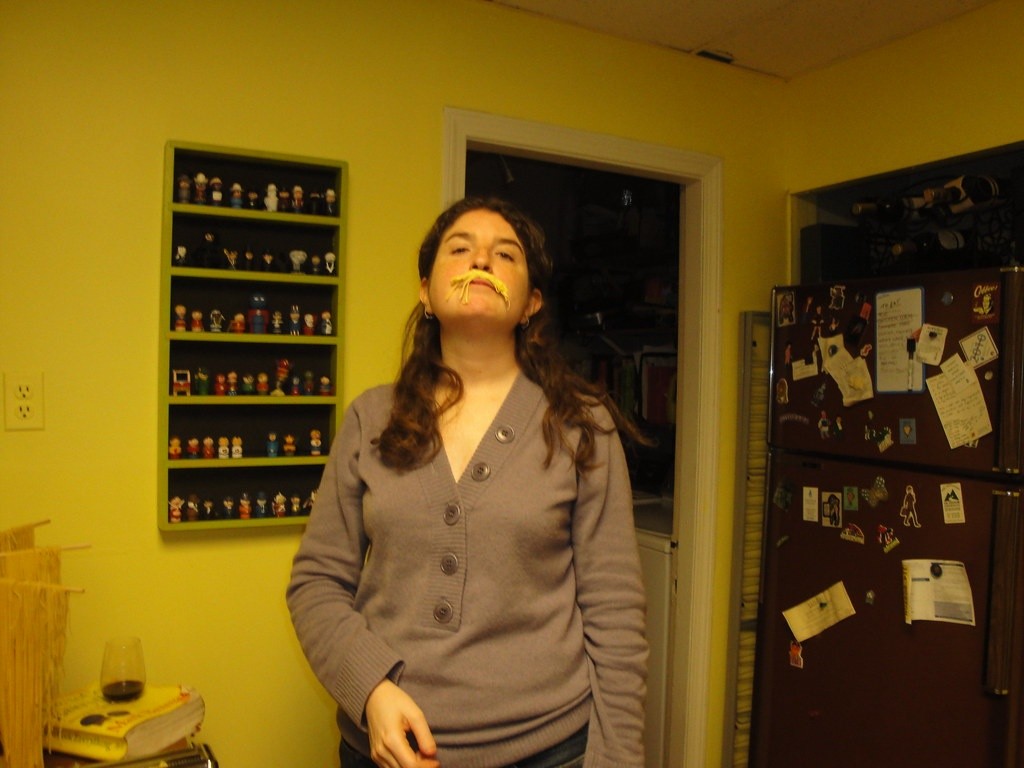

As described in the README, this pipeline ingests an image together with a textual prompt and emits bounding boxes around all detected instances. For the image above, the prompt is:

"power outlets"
[3,370,45,431]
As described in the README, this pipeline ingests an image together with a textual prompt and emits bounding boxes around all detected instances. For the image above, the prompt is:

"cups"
[101,636,145,703]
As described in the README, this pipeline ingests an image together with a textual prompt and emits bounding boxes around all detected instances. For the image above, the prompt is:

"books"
[44,684,211,768]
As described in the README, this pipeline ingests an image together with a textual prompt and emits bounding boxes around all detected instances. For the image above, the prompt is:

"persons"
[278,186,290,211]
[202,436,214,459]
[175,305,332,336]
[287,197,646,768]
[168,494,234,523]
[308,189,336,216]
[209,177,222,205]
[174,232,336,276]
[178,174,191,204]
[291,186,304,212]
[230,183,242,208]
[194,173,208,204]
[187,436,199,459]
[248,188,258,208]
[264,184,278,212]
[231,436,242,459]
[267,432,295,457]
[169,437,181,459]
[218,435,229,459]
[239,488,317,520]
[193,368,332,395]
[310,430,321,456]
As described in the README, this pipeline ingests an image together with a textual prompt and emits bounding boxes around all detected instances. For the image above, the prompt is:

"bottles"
[854,163,1023,258]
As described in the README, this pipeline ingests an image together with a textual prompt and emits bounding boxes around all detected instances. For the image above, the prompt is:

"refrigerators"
[746,267,1024,768]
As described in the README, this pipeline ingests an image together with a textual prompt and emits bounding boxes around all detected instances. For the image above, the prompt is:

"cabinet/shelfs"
[157,140,349,534]
